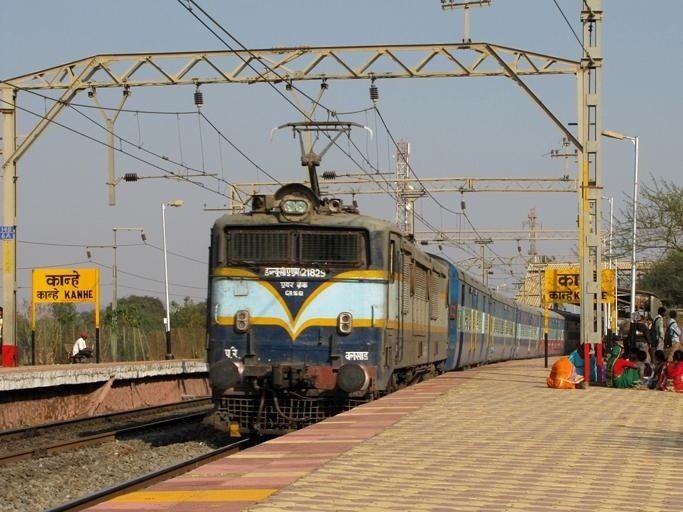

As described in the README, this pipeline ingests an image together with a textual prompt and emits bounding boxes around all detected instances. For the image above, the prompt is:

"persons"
[545,304,683,392]
[0,306,3,355]
[70,331,94,363]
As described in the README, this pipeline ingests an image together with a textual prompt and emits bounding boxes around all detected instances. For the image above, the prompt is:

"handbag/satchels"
[647,380,658,389]
[547,356,585,389]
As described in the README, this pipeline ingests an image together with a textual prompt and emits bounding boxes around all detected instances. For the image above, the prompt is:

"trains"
[206,120,582,436]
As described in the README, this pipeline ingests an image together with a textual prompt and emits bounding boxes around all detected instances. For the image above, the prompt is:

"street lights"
[598,129,641,328]
[161,198,184,360]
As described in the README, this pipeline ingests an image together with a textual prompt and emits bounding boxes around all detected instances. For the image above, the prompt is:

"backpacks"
[663,322,678,349]
[645,317,661,347]
